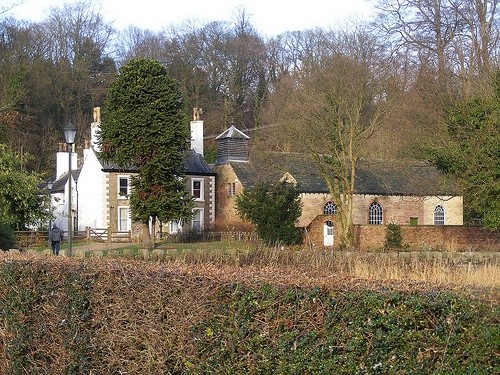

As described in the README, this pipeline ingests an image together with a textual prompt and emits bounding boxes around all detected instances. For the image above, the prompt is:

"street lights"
[46,180,54,241]
[62,122,78,257]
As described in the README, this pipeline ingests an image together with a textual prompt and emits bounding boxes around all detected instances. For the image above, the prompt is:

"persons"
[48,223,64,257]
